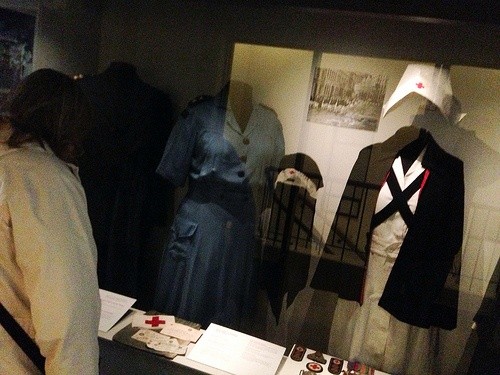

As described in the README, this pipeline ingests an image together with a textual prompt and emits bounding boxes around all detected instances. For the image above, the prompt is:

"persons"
[310,126,464,374]
[153,79,284,332]
[0,64,101,375]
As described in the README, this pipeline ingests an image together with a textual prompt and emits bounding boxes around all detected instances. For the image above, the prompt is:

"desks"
[95,306,388,375]
